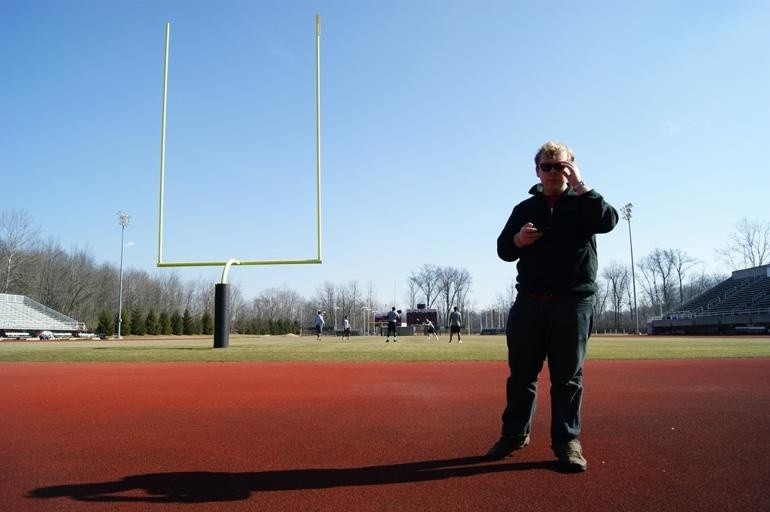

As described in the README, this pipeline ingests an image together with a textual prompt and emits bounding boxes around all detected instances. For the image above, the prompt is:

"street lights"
[497,305,501,328]
[333,306,339,328]
[117,210,132,337]
[619,203,639,334]
[361,307,378,336]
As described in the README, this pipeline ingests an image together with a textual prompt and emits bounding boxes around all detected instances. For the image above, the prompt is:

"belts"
[526,293,570,301]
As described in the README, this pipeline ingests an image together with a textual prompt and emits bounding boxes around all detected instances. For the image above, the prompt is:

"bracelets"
[572,179,586,192]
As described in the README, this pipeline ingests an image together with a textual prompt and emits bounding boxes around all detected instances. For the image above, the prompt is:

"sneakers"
[554,438,587,472]
[487,434,530,459]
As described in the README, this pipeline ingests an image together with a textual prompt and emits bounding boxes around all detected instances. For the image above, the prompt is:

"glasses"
[539,162,568,172]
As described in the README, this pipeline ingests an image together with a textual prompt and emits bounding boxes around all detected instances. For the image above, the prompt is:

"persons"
[313,310,326,340]
[421,317,440,340]
[484,141,619,472]
[342,313,352,341]
[449,306,463,343]
[386,306,398,342]
